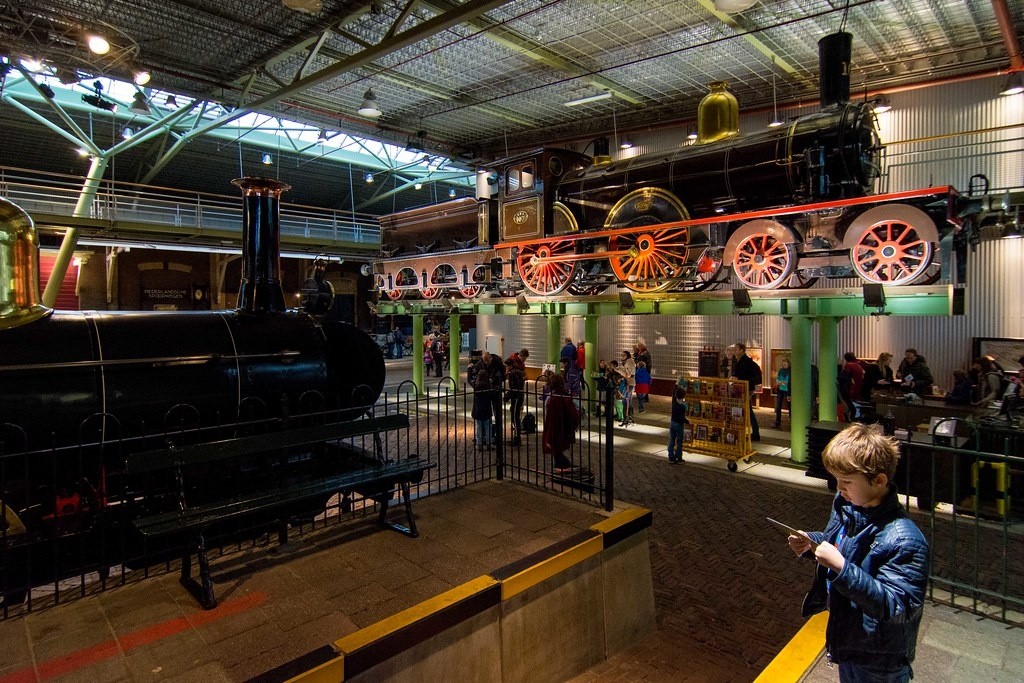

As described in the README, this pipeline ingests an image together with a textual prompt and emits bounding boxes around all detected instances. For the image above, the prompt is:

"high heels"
[619,421,628,428]
[627,419,634,426]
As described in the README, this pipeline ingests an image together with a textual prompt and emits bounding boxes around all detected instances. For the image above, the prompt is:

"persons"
[771,348,1024,429]
[788,421,931,682]
[720,342,762,441]
[592,339,652,427]
[386,326,404,359]
[467,337,589,469]
[423,329,450,377]
[667,375,693,464]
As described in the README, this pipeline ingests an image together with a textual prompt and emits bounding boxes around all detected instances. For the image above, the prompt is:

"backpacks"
[747,360,763,385]
[998,377,1021,401]
[521,412,535,434]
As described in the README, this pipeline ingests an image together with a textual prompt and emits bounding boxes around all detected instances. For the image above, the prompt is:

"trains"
[1,177,411,614]
[373,32,1007,302]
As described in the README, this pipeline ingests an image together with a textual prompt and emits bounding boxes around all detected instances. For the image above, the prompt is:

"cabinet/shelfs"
[674,375,759,472]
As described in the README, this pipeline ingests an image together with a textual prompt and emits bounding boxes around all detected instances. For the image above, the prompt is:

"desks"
[869,393,1004,434]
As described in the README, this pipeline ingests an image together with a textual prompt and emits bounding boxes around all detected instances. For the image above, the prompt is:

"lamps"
[414,182,422,190]
[620,137,633,149]
[405,134,426,154]
[563,92,612,108]
[478,164,487,174]
[261,152,274,166]
[767,111,785,126]
[869,93,893,114]
[999,72,1024,96]
[686,125,698,140]
[0,26,151,158]
[363,170,375,182]
[318,129,329,142]
[357,87,382,118]
[448,189,457,198]
[423,155,431,164]
[164,95,180,110]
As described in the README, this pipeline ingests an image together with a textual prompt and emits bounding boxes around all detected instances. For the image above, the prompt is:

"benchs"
[124,413,438,611]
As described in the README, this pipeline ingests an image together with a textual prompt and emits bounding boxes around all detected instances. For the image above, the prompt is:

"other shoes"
[746,433,760,441]
[770,422,782,427]
[675,459,686,464]
[669,459,678,464]
